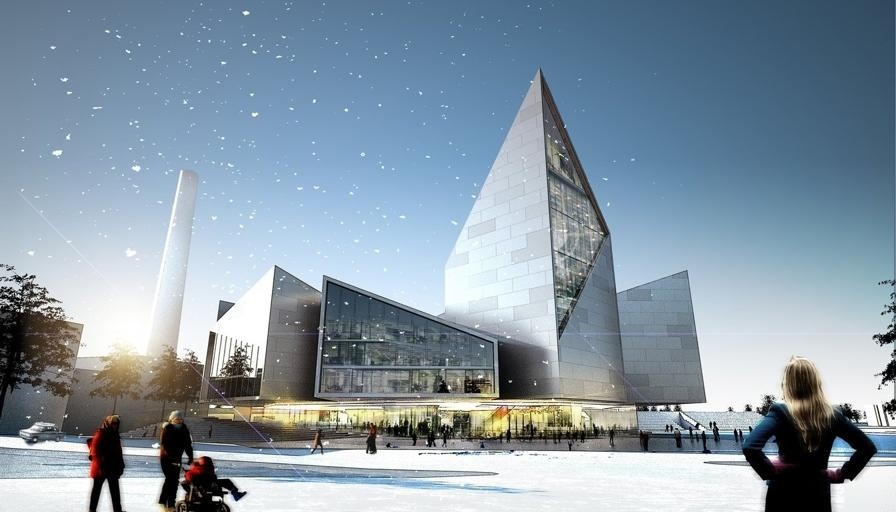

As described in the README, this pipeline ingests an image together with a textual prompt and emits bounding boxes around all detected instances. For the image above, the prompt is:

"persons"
[740,355,877,512]
[387,418,455,447]
[665,421,753,451]
[639,430,649,452]
[152,425,157,437]
[156,410,194,512]
[345,418,377,454]
[183,455,248,502]
[310,429,324,455]
[208,422,213,438]
[526,421,616,451]
[87,414,127,512]
[499,429,511,443]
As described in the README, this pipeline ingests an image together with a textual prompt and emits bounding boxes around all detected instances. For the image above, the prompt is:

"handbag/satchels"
[86,438,94,461]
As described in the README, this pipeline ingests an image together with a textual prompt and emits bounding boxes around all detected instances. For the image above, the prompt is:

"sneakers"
[234,491,247,501]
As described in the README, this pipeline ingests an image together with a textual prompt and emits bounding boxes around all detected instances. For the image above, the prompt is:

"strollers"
[169,461,231,512]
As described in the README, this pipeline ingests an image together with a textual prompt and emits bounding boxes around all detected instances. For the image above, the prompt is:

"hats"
[316,428,323,432]
[168,410,184,419]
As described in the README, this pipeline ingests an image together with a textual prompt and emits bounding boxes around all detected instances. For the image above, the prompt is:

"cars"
[20,423,64,442]
